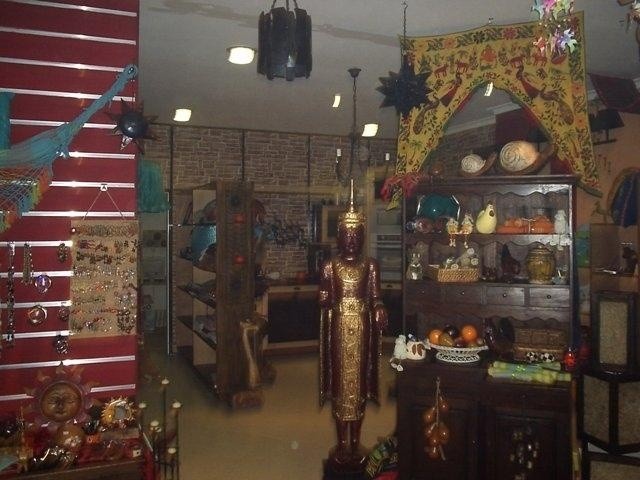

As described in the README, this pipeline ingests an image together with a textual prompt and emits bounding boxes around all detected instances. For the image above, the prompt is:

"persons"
[317,179,389,466]
[41,383,79,422]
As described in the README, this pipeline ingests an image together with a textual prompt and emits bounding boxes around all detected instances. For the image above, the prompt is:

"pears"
[439,332,455,346]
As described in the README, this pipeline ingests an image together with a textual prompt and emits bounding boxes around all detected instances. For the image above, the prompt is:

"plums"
[444,326,460,339]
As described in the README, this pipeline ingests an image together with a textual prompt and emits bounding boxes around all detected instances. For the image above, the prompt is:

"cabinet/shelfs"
[167,179,259,412]
[390,174,584,480]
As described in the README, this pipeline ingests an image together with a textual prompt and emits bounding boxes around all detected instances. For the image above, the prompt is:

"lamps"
[335,68,390,189]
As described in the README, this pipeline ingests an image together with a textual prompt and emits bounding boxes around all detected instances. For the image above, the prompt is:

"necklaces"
[6,243,15,348]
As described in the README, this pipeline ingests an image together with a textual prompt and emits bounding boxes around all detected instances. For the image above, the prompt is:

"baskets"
[427,265,481,283]
[426,339,489,363]
